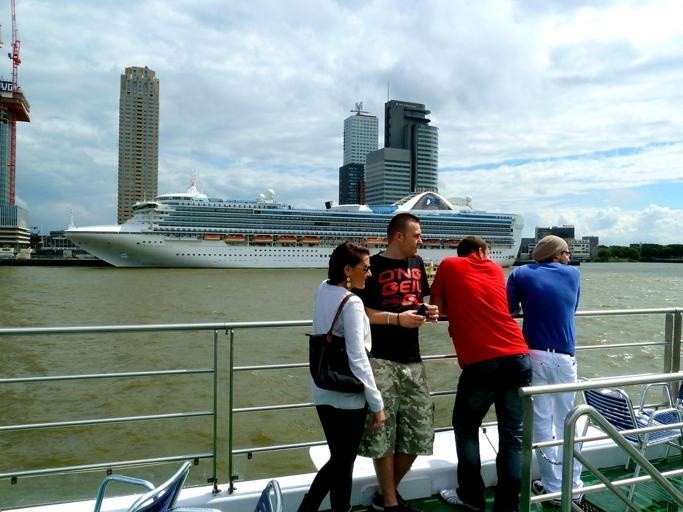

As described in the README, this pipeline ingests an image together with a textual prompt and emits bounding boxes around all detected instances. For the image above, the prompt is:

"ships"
[63,168,525,269]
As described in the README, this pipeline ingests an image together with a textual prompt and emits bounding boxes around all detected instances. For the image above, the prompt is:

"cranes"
[6,0,22,204]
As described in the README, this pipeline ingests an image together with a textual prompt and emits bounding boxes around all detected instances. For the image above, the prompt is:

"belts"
[528,345,576,358]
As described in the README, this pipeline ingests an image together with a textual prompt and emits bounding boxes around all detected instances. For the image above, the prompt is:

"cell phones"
[416,304,428,315]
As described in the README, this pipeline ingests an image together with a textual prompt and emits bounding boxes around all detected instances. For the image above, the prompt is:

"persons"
[430,234,533,512]
[349,212,439,512]
[502,235,586,506]
[294,239,387,512]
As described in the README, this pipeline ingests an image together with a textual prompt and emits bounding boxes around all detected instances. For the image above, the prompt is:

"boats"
[422,258,439,288]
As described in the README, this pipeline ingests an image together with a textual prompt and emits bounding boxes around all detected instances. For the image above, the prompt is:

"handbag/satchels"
[301,332,371,394]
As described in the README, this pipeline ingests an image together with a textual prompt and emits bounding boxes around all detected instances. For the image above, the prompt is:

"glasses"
[356,265,371,273]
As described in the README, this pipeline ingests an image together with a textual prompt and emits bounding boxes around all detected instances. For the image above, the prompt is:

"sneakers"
[568,485,587,504]
[532,479,563,506]
[371,488,416,510]
[438,487,487,510]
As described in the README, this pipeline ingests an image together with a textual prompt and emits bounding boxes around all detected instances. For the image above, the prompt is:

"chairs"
[573,372,682,512]
[252,479,284,512]
[624,379,682,471]
[91,459,192,512]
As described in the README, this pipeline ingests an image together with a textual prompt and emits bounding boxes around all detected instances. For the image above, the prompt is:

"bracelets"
[386,310,392,325]
[396,312,401,327]
